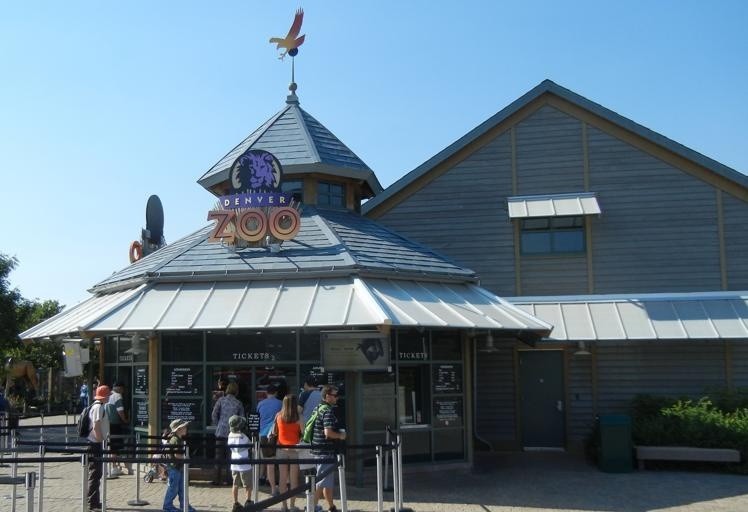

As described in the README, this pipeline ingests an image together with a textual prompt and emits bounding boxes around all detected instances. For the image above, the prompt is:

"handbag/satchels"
[261,434,276,457]
[303,415,317,443]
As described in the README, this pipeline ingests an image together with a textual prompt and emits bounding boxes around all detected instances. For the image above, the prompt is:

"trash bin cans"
[598,413,633,473]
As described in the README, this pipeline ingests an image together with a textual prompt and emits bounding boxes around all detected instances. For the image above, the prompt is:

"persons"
[105,383,130,475]
[258,384,284,497]
[268,394,304,510]
[93,377,100,402]
[163,419,197,511]
[303,385,346,512]
[211,382,245,486]
[78,387,110,512]
[227,415,254,511]
[297,375,322,434]
[80,379,88,404]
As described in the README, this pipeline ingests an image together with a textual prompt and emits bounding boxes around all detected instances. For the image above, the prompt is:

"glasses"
[328,393,337,398]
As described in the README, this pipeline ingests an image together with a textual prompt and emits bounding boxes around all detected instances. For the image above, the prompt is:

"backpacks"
[76,402,105,437]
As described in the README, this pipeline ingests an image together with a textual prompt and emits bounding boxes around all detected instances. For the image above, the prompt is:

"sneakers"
[112,467,124,475]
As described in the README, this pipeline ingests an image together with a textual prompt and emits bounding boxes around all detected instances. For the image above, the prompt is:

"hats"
[93,385,113,400]
[113,380,124,388]
[229,415,246,433]
[170,419,189,433]
